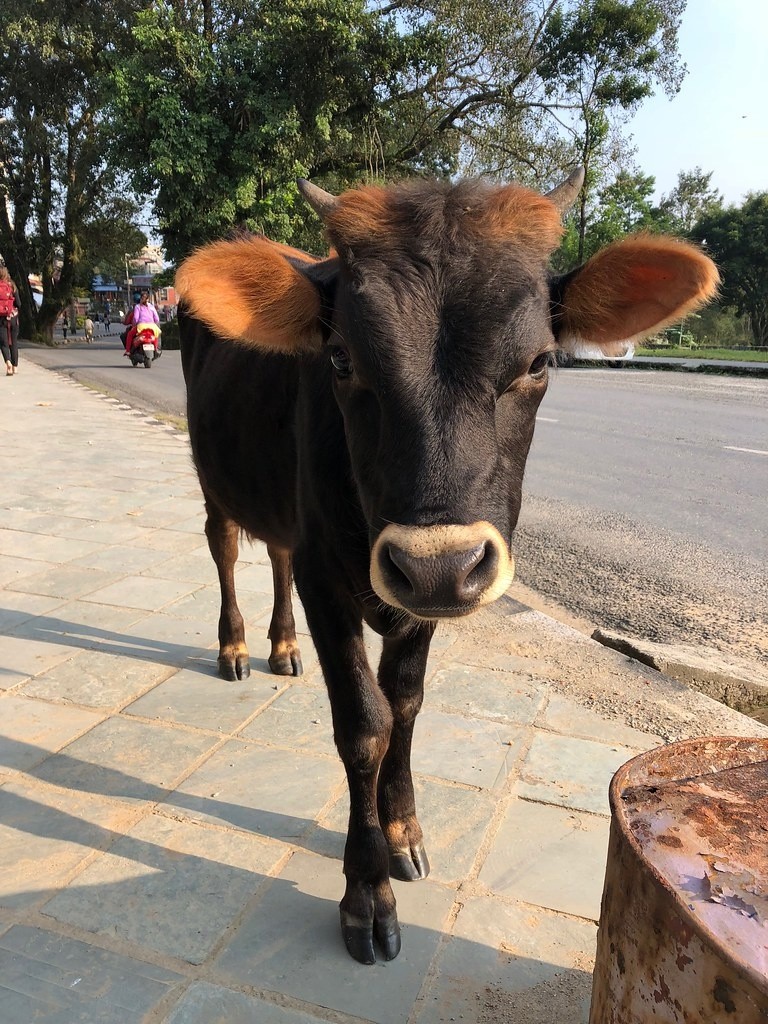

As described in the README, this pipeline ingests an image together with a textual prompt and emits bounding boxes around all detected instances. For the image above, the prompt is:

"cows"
[168,165,724,966]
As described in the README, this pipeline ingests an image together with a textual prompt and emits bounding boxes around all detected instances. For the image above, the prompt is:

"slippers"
[6,373,13,376]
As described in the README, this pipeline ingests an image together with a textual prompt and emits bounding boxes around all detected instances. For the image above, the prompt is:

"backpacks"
[0,280,16,317]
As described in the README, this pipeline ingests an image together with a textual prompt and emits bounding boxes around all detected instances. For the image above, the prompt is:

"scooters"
[125,327,161,368]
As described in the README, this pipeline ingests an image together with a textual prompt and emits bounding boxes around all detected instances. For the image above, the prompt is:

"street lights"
[124,253,131,307]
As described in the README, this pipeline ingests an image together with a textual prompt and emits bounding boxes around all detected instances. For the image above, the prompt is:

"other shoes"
[123,352,130,356]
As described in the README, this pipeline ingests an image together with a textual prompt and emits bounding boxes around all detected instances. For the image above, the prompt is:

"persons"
[84,316,94,343]
[120,291,162,355]
[0,265,22,375]
[104,313,110,332]
[62,316,69,339]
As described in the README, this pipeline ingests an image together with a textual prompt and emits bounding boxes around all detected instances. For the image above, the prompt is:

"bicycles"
[85,330,94,344]
